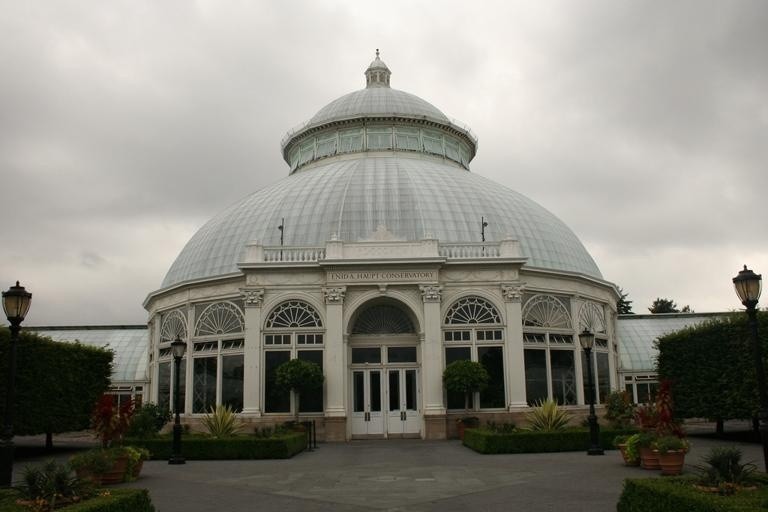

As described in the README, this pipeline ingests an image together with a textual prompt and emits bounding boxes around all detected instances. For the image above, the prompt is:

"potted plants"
[442,359,492,441]
[612,432,641,466]
[653,435,693,475]
[67,444,144,484]
[637,432,663,470]
[275,358,324,433]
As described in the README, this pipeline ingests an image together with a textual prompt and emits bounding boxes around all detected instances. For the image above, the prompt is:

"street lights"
[579,328,605,456]
[1,280,32,486]
[170,336,186,465]
[733,265,767,474]
